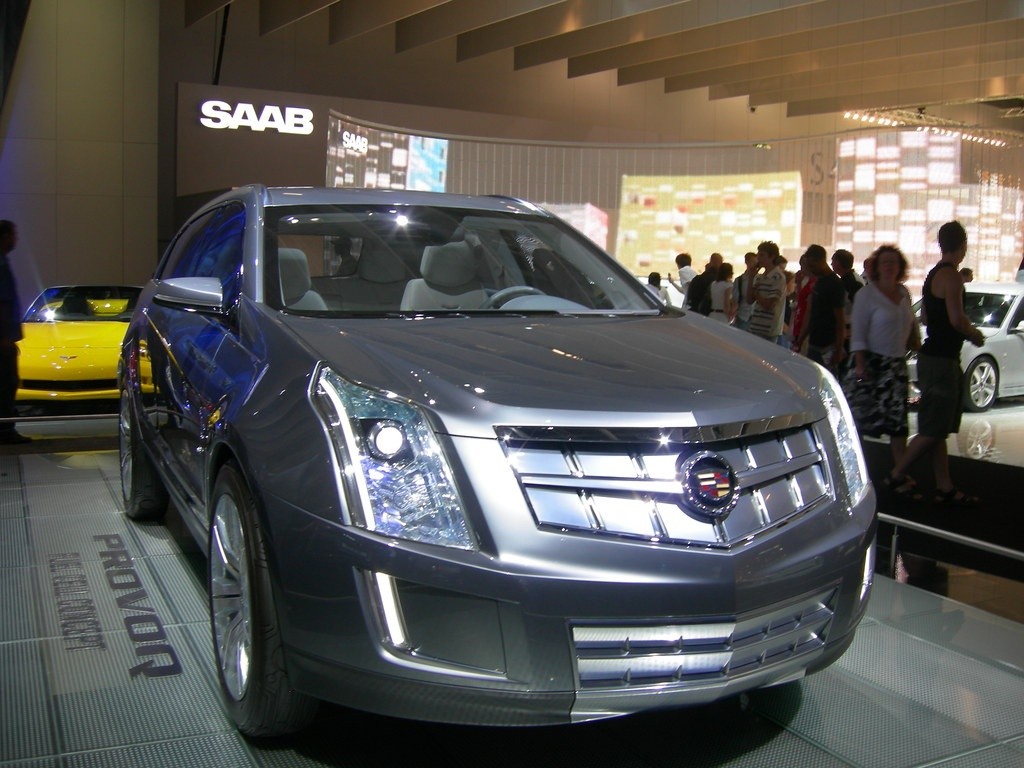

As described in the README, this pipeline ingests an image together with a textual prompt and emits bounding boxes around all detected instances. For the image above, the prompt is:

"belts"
[710,310,724,313]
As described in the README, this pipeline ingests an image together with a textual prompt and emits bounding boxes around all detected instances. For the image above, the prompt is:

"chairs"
[278,239,489,311]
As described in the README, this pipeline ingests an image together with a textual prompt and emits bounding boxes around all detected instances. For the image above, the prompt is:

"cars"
[11,286,143,440]
[904,280,1024,412]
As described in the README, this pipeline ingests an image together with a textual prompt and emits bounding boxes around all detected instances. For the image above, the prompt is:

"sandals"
[883,473,928,503]
[932,485,979,508]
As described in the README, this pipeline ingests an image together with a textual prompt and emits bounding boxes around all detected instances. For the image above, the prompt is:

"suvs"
[116,184,878,744]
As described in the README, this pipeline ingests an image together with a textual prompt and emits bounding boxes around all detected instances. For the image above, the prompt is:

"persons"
[883,222,985,504]
[646,241,916,485]
[329,235,357,277]
[0,220,22,445]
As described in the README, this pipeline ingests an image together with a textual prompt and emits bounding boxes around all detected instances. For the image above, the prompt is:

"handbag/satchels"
[905,292,922,351]
